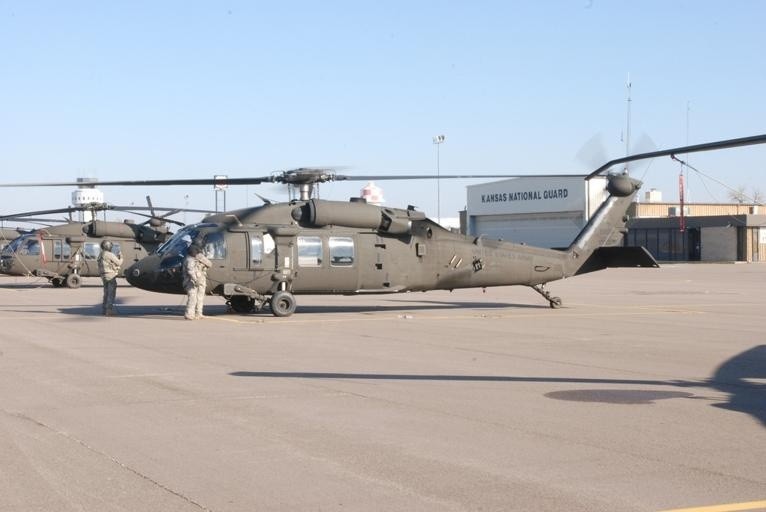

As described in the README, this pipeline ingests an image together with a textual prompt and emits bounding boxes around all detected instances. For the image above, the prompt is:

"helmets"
[187,237,204,253]
[101,240,113,251]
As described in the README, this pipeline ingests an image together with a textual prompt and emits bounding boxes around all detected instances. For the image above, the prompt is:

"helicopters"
[0,201,225,289]
[0,168,660,318]
[0,215,54,257]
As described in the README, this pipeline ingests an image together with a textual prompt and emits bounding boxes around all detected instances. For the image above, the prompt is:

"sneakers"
[101,306,123,318]
[185,314,209,321]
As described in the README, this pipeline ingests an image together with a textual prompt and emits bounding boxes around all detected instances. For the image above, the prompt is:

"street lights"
[432,134,446,229]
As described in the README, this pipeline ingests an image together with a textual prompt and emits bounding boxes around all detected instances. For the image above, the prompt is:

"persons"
[192,241,212,319]
[96,239,124,316]
[181,246,203,321]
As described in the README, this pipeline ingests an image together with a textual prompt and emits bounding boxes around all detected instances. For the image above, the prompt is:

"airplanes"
[585,133,766,185]
